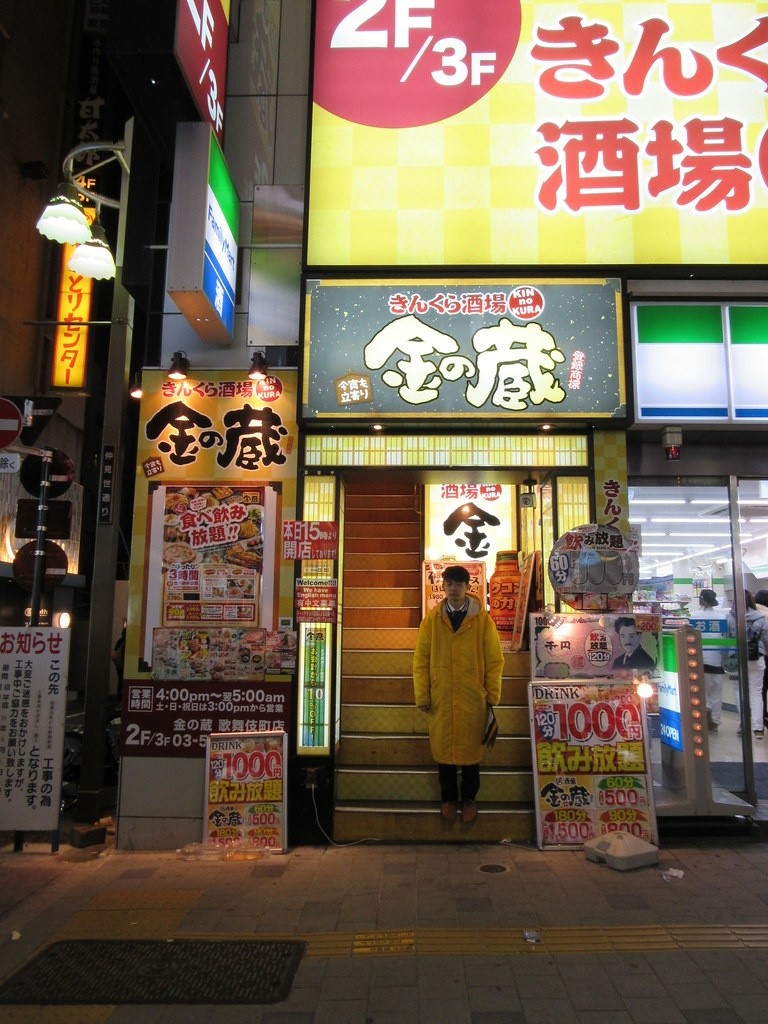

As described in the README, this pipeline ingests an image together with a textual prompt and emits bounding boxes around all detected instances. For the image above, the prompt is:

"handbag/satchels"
[112,647,125,667]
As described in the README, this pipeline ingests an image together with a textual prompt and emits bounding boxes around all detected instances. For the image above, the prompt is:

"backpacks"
[730,612,764,661]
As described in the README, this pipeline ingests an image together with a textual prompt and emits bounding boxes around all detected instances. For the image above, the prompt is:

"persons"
[691,589,736,732]
[731,589,768,739]
[413,566,505,822]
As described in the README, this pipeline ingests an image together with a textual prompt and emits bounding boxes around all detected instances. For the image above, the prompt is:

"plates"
[238,580,248,586]
[228,587,241,597]
[242,586,254,596]
[489,551,522,652]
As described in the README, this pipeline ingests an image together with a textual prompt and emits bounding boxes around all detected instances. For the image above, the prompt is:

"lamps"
[36,142,129,280]
[248,349,269,379]
[167,350,189,379]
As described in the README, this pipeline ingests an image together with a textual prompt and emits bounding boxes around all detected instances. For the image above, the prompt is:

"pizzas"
[163,545,196,564]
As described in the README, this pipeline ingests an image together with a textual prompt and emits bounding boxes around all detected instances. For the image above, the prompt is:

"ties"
[451,611,460,630]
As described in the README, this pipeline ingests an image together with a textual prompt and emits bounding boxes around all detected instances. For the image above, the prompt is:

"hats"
[700,590,718,606]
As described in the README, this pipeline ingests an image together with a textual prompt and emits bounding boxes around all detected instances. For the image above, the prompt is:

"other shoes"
[441,802,457,822]
[736,725,742,736]
[462,801,478,823]
[754,729,764,738]
[712,723,719,732]
